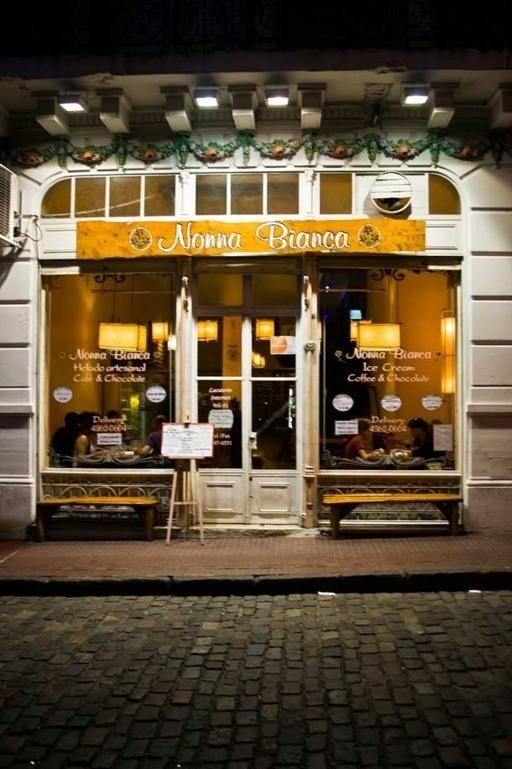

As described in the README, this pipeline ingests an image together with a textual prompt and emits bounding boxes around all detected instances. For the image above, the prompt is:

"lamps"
[263,83,292,109]
[96,275,147,353]
[355,322,402,351]
[198,318,218,343]
[438,315,458,401]
[399,81,431,108]
[151,320,168,344]
[256,318,275,339]
[193,85,222,111]
[252,350,266,370]
[58,90,90,115]
[349,319,372,344]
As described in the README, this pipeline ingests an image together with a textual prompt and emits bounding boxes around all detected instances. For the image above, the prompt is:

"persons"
[417,418,446,459]
[407,415,430,455]
[344,419,375,461]
[132,413,167,456]
[50,409,130,465]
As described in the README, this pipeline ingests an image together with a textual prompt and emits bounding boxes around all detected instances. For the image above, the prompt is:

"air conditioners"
[0,159,24,253]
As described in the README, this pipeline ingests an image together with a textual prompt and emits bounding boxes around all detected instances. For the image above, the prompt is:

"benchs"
[318,489,460,539]
[34,493,165,551]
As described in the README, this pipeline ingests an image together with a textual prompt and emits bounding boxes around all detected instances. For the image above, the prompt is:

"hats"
[409,417,428,428]
